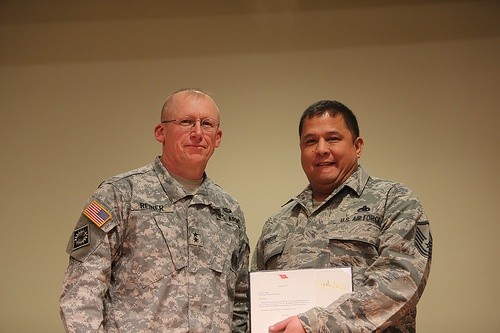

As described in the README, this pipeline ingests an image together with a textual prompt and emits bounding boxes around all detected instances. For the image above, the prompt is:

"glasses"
[160,116,219,131]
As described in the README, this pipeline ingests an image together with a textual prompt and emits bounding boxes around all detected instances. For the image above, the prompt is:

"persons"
[250,100,433,333]
[58,88,250,333]
